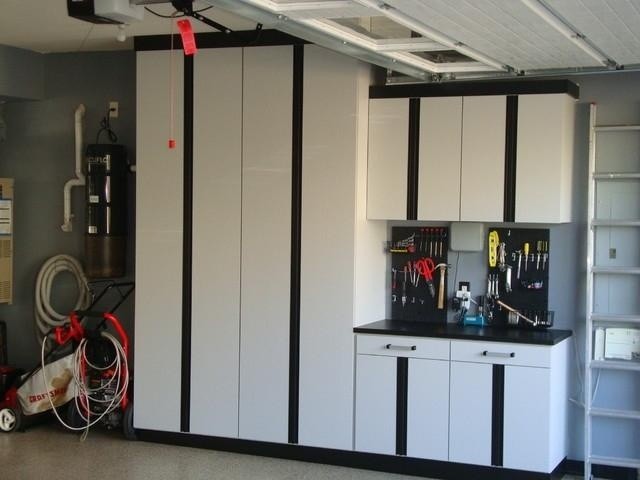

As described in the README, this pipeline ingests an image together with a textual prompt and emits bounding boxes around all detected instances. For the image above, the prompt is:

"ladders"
[584,101,640,480]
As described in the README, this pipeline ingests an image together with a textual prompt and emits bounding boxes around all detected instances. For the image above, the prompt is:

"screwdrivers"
[404,261,421,287]
[524,240,547,271]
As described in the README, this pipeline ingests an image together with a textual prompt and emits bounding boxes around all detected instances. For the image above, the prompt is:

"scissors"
[415,257,436,298]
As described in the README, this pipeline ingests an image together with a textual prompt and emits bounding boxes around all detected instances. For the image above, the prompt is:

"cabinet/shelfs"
[448,339,572,476]
[368,96,461,224]
[355,332,449,466]
[133,44,242,453]
[239,42,390,458]
[463,96,578,227]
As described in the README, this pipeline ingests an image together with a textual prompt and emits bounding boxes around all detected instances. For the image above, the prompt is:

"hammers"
[435,263,452,310]
